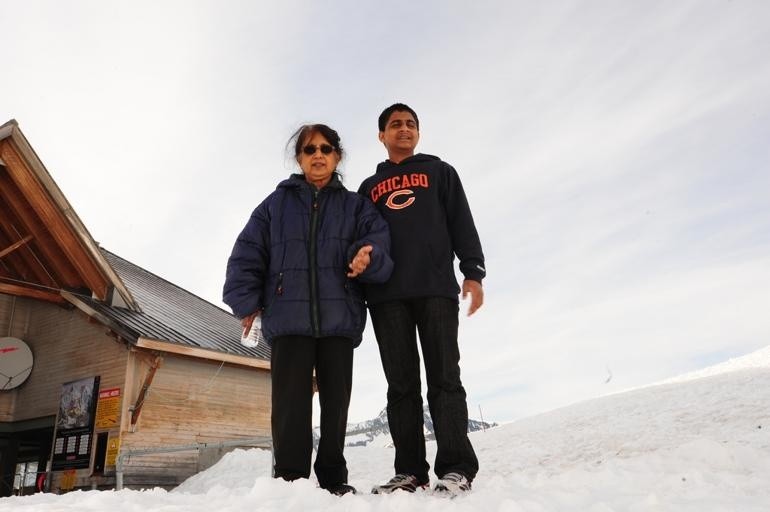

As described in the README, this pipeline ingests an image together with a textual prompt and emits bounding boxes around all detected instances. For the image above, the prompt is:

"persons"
[222,122,395,497]
[356,103,487,499]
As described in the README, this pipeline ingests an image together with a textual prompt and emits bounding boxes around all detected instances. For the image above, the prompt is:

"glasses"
[300,143,336,154]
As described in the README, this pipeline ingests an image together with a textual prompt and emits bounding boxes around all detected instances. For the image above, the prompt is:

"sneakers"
[432,473,473,498]
[318,483,358,498]
[369,471,430,496]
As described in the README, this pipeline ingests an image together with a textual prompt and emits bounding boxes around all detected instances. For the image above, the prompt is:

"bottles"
[240,310,262,349]
